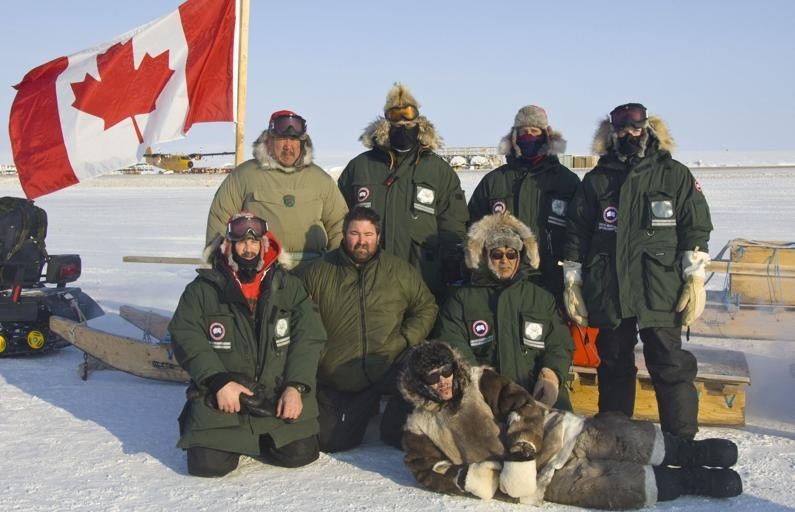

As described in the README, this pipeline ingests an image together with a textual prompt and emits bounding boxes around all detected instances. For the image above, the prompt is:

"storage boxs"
[728,241,795,309]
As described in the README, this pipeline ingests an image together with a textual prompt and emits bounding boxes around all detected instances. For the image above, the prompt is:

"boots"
[660,438,738,468]
[652,465,743,501]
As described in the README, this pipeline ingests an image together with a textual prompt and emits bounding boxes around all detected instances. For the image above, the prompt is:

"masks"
[391,123,419,148]
[517,134,547,157]
[616,133,641,153]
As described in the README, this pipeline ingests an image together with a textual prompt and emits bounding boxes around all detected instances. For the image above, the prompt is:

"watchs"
[288,382,308,393]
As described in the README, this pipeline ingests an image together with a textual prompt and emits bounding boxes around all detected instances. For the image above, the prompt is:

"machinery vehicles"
[0,253,105,358]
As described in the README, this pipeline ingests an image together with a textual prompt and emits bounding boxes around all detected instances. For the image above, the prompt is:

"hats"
[220,213,270,272]
[269,110,304,133]
[497,105,567,156]
[357,83,434,150]
[486,223,523,251]
[408,343,460,402]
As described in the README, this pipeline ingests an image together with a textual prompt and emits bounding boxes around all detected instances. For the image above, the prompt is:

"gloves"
[556,260,589,329]
[500,458,538,499]
[464,461,501,501]
[675,245,711,327]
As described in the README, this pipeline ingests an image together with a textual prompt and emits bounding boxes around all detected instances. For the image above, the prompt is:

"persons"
[396,337,744,509]
[438,210,576,416]
[466,102,581,322]
[560,101,714,436]
[165,211,328,476]
[336,82,469,310]
[203,106,352,273]
[298,208,440,451]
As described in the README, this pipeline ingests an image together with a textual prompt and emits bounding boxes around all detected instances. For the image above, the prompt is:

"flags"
[6,0,241,201]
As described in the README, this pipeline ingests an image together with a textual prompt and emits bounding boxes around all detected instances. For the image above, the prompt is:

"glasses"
[610,103,648,128]
[387,105,418,122]
[422,366,453,385]
[491,250,517,261]
[227,217,268,237]
[273,116,307,135]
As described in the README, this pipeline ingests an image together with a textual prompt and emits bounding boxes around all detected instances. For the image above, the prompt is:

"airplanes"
[143,146,236,174]
